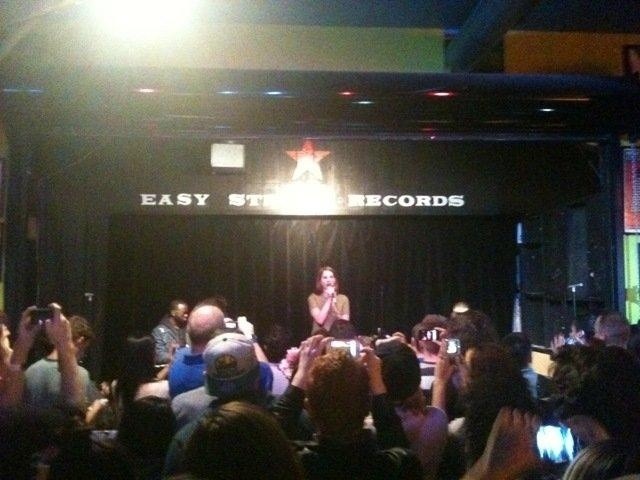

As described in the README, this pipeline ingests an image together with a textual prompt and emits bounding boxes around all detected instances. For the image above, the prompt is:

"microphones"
[328,284,337,304]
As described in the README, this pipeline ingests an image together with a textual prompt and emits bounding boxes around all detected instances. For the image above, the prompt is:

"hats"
[202,332,257,381]
[375,341,420,397]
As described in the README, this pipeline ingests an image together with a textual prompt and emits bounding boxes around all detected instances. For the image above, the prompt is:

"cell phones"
[418,329,438,342]
[532,423,582,465]
[31,308,54,325]
[445,339,461,356]
[327,339,361,358]
[223,321,239,332]
[565,336,578,346]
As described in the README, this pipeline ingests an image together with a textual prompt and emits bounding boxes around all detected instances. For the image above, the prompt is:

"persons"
[306,266,350,336]
[0,296,638,478]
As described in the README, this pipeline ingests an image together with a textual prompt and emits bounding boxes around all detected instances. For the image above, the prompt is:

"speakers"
[211,143,246,169]
[443,28,505,73]
